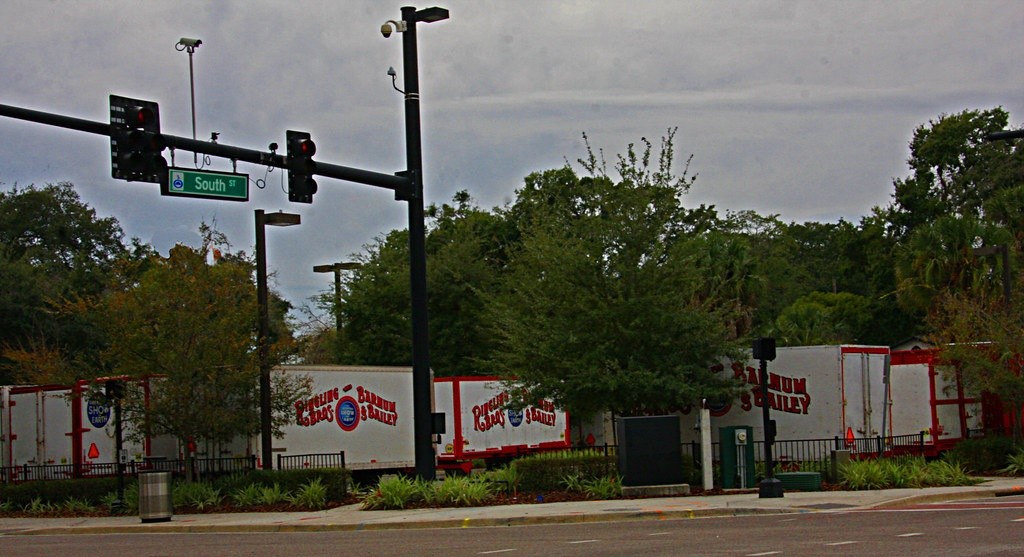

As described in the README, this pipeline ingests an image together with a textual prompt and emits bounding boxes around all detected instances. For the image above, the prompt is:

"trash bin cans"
[136,456,174,524]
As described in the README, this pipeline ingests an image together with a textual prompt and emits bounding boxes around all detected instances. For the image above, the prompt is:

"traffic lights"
[109,94,168,183]
[105,378,126,399]
[381,6,451,480]
[285,129,318,205]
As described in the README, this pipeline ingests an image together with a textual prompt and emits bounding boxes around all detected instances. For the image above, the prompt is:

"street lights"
[254,207,301,471]
[312,261,362,333]
